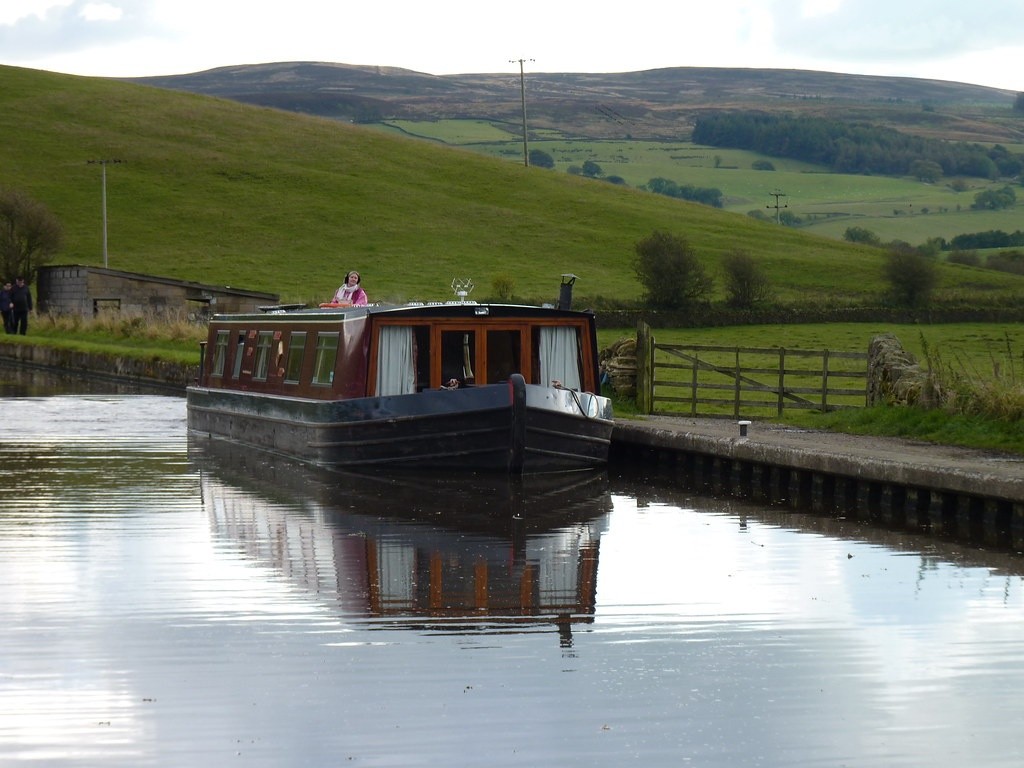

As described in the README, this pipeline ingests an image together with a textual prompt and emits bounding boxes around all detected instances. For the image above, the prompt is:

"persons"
[319,270,367,307]
[0,282,13,334]
[8,276,32,336]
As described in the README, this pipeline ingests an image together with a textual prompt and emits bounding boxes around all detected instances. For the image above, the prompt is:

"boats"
[183,431,614,653]
[188,272,618,479]
